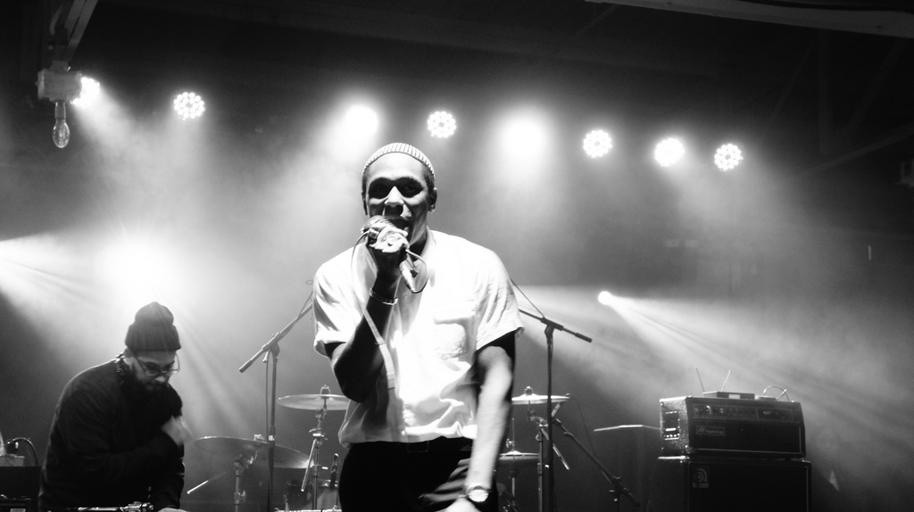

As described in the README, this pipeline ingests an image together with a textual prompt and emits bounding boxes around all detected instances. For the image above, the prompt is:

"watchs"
[464,486,495,504]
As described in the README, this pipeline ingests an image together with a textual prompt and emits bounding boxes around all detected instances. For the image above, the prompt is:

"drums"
[284,477,343,512]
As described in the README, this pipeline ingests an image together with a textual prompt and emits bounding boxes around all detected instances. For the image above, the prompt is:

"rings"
[387,242,393,246]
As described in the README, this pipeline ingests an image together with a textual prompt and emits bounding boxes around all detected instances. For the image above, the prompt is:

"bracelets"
[368,287,399,307]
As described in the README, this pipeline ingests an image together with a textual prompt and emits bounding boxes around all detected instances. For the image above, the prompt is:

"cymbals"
[276,393,351,411]
[497,451,539,464]
[512,393,571,406]
[193,435,316,470]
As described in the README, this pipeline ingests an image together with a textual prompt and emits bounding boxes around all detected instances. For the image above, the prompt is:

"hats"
[125,302,182,352]
[362,142,435,178]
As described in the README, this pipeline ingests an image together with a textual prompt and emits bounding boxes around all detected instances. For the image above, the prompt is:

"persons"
[315,142,525,512]
[38,301,193,512]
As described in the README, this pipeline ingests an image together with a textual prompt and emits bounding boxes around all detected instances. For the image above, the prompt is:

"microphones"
[536,400,566,441]
[363,214,417,293]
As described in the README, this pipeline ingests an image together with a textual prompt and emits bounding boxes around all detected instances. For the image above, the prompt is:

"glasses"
[130,348,181,376]
